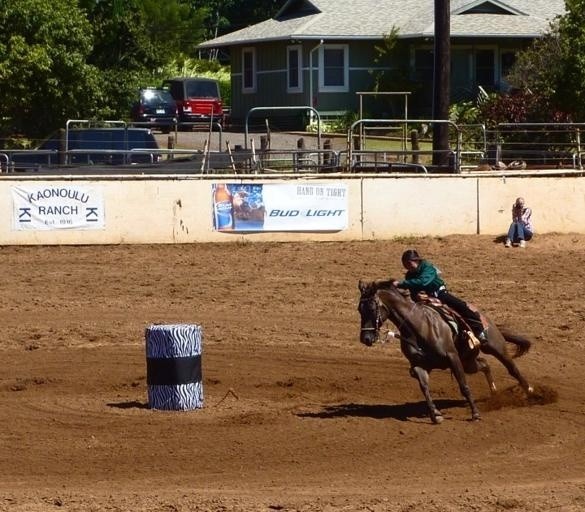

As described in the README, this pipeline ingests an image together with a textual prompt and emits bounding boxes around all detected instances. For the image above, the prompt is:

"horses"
[357,278,535,425]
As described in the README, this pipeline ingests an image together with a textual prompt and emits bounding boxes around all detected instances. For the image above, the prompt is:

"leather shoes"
[480,332,487,343]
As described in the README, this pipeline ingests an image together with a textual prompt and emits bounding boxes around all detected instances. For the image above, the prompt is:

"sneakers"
[505,239,511,247]
[520,240,525,248]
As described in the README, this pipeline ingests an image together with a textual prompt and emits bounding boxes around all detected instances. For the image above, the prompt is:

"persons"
[504,197,534,248]
[391,249,489,347]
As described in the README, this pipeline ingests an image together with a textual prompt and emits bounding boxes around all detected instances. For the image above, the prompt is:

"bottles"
[213,183,233,230]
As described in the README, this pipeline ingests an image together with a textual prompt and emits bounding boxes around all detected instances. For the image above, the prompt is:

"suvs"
[163,78,223,132]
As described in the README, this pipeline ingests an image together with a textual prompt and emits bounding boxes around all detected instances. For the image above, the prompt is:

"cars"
[0,127,162,165]
[130,86,179,134]
